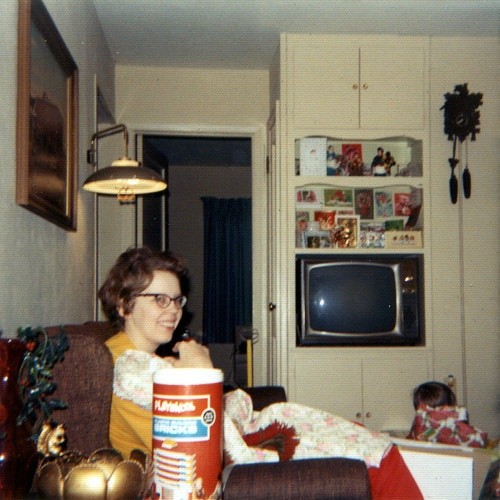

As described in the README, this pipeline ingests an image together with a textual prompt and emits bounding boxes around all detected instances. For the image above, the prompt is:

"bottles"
[372,149,388,176]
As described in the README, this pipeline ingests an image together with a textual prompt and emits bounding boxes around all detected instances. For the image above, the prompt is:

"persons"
[97,243,213,461]
[327,145,396,176]
[413,381,500,451]
[354,190,373,219]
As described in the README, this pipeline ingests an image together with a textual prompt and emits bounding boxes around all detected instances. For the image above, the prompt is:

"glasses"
[132,294,187,308]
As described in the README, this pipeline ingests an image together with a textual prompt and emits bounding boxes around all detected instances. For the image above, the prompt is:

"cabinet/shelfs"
[270,33,500,437]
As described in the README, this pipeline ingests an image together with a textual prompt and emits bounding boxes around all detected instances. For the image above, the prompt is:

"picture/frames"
[301,229,332,249]
[16,0,80,232]
[335,215,360,248]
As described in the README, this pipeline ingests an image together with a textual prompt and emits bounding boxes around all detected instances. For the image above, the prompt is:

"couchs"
[14,321,372,500]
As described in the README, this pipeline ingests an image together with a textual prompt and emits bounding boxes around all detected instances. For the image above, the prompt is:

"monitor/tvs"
[294,253,426,346]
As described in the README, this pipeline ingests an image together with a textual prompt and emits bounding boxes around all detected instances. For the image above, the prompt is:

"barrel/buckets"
[152,367,225,500]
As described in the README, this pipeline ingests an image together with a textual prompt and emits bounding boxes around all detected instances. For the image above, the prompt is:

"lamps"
[81,124,167,202]
